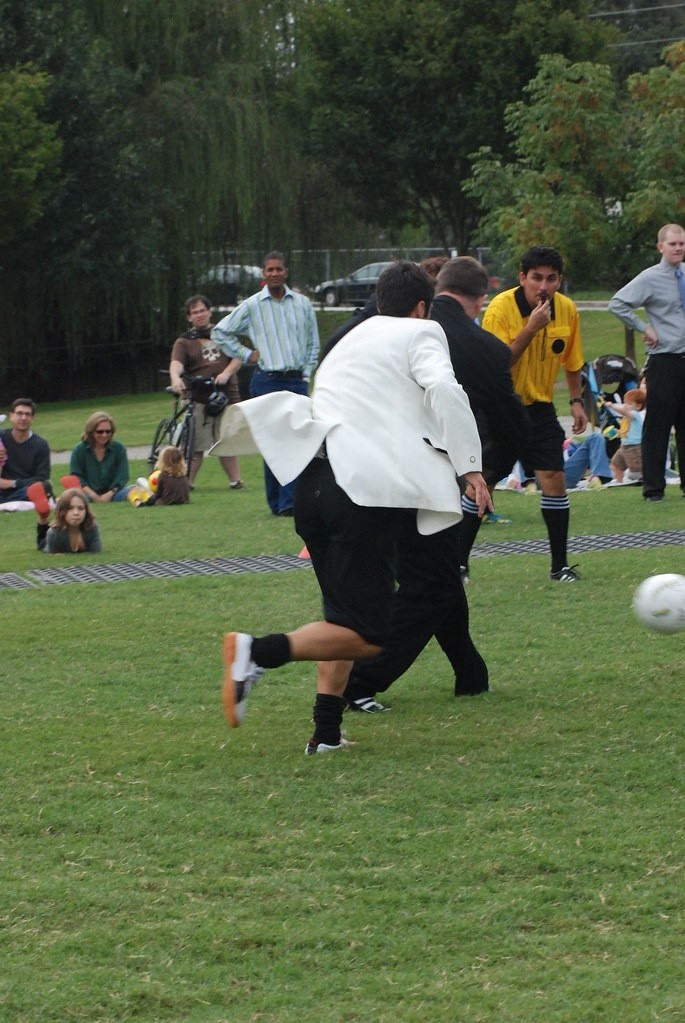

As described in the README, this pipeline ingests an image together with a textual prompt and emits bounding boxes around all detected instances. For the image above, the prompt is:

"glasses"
[14,410,32,416]
[188,308,208,314]
[94,429,113,434]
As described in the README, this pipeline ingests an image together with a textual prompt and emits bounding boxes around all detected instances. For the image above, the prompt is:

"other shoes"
[230,480,245,489]
[483,512,510,525]
[60,475,82,491]
[27,482,50,518]
[644,495,662,503]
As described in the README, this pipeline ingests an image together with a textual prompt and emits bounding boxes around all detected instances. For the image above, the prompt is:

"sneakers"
[344,696,393,713]
[549,564,581,583]
[221,631,266,727]
[304,738,362,756]
[460,565,470,585]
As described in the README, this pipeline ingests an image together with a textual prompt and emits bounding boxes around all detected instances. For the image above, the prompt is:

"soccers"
[632,573,685,635]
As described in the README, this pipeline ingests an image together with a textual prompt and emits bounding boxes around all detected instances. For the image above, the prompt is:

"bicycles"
[147,374,227,484]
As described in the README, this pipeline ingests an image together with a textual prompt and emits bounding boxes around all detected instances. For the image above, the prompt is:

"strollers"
[586,354,643,457]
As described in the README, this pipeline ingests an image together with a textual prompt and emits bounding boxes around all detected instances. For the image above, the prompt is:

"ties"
[675,269,685,313]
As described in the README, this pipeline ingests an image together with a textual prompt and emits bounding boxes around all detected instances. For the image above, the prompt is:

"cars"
[195,264,292,309]
[311,260,422,307]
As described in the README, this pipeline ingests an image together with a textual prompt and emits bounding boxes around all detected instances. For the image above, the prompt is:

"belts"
[272,369,302,377]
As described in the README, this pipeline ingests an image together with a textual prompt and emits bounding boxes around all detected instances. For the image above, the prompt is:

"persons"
[608,221,685,503]
[210,251,322,516]
[26,476,102,553]
[147,446,190,505]
[169,295,245,492]
[207,260,496,763]
[603,373,648,482]
[323,246,583,713]
[71,411,138,501]
[0,398,50,503]
[516,434,613,494]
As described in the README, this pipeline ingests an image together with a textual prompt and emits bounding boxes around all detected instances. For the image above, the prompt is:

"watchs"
[569,397,584,408]
